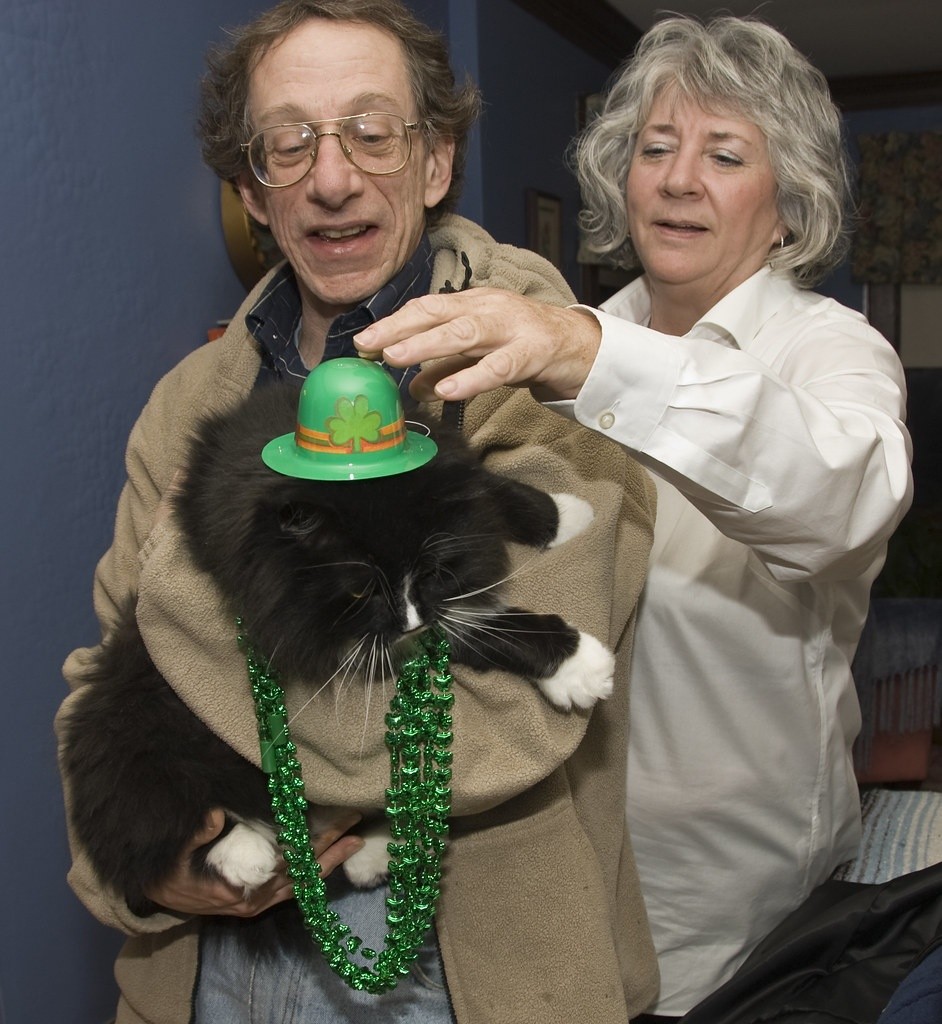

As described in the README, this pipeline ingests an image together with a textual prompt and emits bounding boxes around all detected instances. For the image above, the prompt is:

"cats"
[56,367,619,898]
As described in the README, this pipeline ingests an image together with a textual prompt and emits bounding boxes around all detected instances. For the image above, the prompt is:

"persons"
[352,9,914,1024]
[52,0,660,1024]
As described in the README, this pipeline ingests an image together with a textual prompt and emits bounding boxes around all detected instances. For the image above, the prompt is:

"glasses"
[240,112,421,188]
